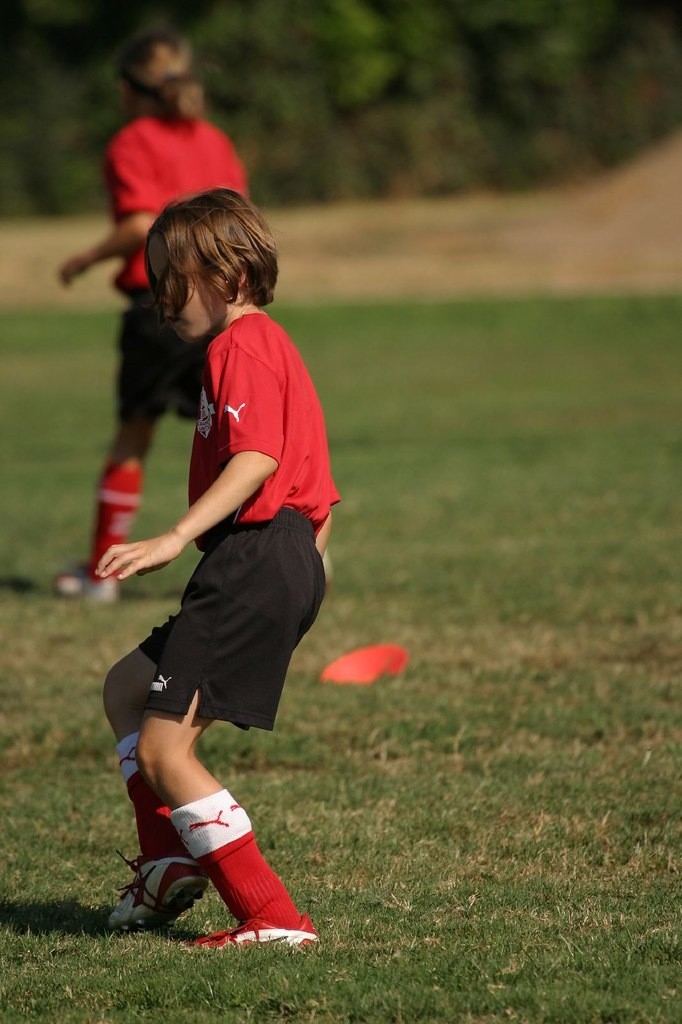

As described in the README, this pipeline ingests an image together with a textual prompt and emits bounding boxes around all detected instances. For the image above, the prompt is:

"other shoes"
[56,574,117,604]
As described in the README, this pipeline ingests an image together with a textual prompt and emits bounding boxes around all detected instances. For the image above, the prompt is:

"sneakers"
[176,912,320,953]
[108,845,209,933]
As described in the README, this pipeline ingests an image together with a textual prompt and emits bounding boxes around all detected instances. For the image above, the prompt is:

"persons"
[95,184,341,954]
[54,32,247,600]
